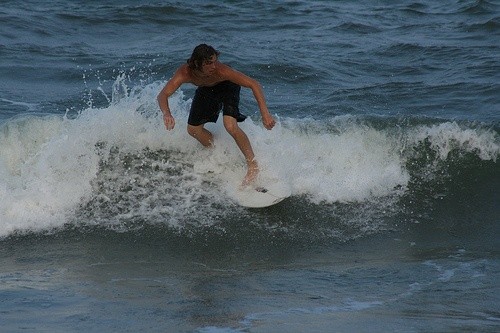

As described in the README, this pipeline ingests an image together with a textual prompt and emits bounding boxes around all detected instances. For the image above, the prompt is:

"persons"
[156,43,277,187]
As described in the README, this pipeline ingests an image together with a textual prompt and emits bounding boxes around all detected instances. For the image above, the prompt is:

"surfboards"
[195,146,293,208]
